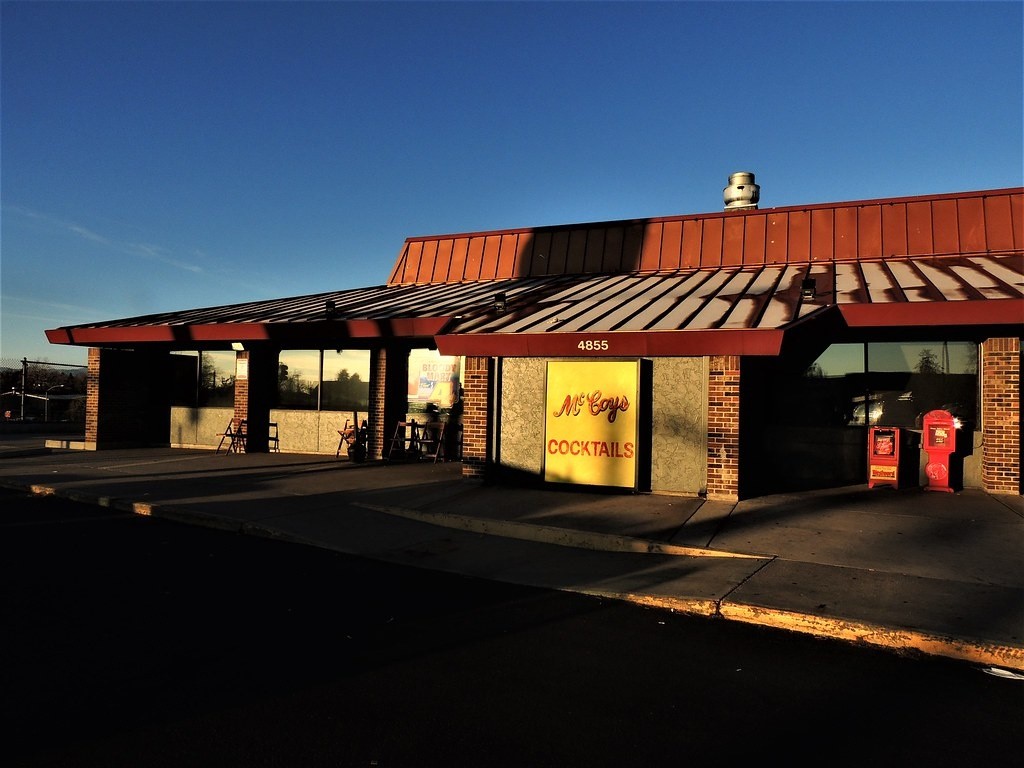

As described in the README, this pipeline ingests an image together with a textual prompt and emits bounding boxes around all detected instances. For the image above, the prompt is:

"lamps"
[325,301,335,318]
[801,278,817,300]
[494,294,506,311]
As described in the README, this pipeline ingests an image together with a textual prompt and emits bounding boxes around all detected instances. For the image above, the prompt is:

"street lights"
[45,384,64,423]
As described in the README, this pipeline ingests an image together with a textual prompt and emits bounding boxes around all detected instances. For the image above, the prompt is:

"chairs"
[335,419,447,465]
[215,418,248,456]
[267,423,279,453]
